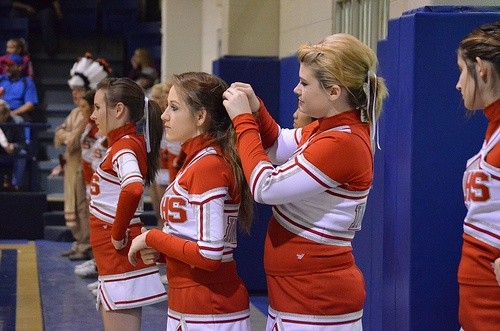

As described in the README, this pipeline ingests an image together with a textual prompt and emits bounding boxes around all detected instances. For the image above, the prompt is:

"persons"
[0,39,39,190]
[456,21,500,331]
[223,34,389,331]
[128,72,258,331]
[55,49,183,296]
[90,78,167,331]
[292,107,316,128]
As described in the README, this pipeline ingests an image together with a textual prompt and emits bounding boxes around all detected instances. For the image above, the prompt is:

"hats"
[8,54,23,64]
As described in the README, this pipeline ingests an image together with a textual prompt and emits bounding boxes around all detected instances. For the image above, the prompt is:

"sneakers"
[60,249,90,260]
[74,259,98,277]
[87,280,98,297]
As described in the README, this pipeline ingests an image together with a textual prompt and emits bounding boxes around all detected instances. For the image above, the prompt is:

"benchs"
[0,0,159,173]
